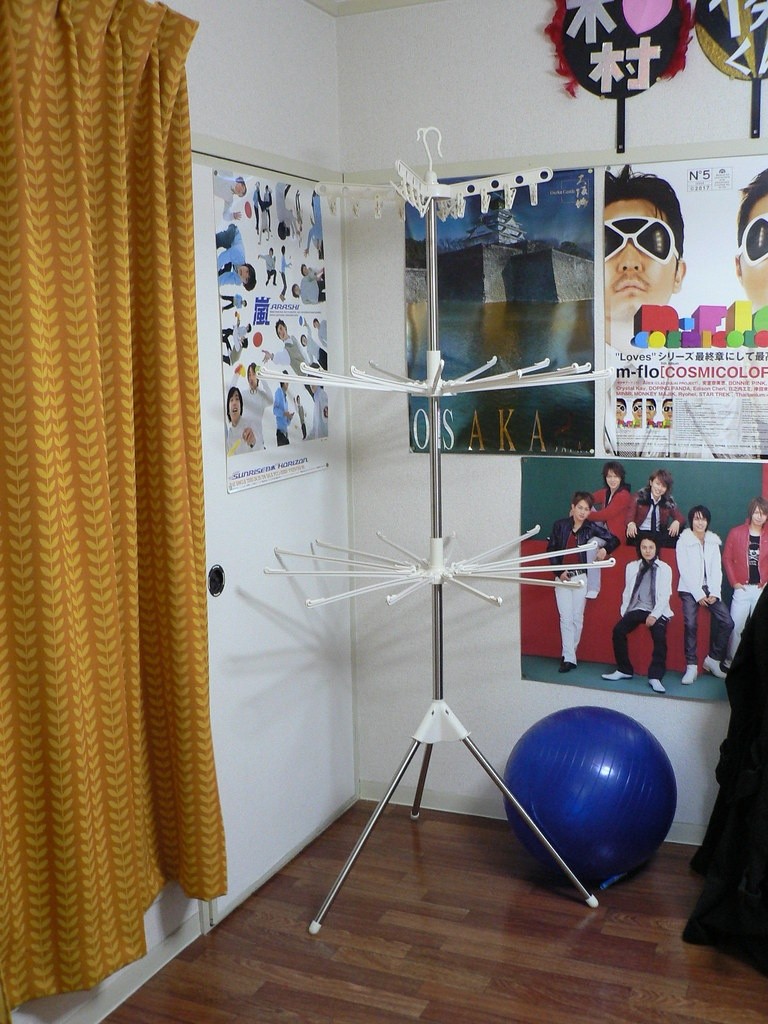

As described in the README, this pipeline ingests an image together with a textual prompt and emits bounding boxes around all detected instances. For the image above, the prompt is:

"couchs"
[520,540,711,676]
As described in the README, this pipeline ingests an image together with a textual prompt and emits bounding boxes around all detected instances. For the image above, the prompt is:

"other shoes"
[559,656,565,664]
[559,662,576,673]
[649,678,666,693]
[681,664,698,684]
[702,655,726,679]
[602,670,633,680]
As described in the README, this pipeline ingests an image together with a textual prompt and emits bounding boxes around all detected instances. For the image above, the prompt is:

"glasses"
[735,214,768,265]
[617,404,625,412]
[647,406,655,411]
[603,218,680,264]
[663,406,672,412]
[632,405,642,413]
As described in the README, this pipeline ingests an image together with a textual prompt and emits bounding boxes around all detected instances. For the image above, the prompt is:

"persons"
[545,462,768,694]
[735,168,768,304]
[601,163,690,322]
[212,171,330,457]
[616,396,672,429]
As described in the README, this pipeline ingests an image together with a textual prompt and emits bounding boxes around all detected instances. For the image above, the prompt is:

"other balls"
[502,706,678,875]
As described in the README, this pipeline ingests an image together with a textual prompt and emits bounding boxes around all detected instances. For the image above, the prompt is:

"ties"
[651,499,661,536]
